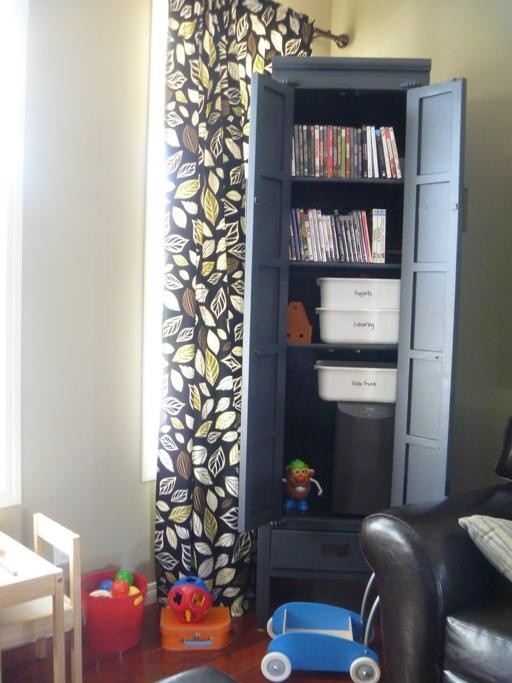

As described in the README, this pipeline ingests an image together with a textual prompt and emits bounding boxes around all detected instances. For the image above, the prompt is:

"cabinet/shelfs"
[236,54,469,623]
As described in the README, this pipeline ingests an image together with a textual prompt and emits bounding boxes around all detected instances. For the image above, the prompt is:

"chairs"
[0,513,83,683]
[357,421,512,683]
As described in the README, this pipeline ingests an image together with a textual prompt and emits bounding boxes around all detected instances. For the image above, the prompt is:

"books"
[288,207,387,265]
[289,124,403,181]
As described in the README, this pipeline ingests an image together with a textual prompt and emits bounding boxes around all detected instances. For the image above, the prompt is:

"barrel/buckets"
[80,566,147,655]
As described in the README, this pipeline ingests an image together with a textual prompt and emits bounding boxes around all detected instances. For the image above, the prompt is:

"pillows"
[459,513,512,586]
